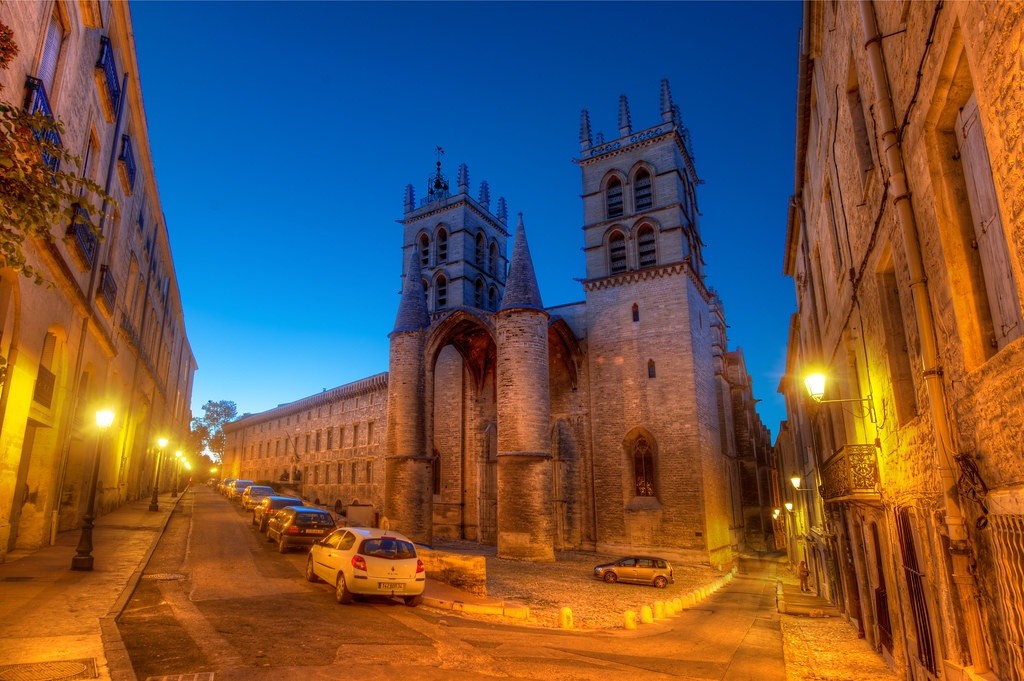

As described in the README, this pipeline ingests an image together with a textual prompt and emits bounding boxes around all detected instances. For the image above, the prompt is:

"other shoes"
[806,589,811,591]
[801,588,804,591]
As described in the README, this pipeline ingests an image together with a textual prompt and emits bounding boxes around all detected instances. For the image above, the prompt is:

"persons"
[798,561,810,591]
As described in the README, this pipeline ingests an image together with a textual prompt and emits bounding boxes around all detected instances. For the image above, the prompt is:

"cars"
[254,495,304,532]
[305,527,426,606]
[266,507,338,553]
[594,555,675,589]
[240,485,277,511]
[207,477,254,502]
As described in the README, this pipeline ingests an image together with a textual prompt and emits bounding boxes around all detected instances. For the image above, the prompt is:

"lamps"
[774,507,787,516]
[785,499,805,513]
[803,374,875,425]
[791,472,817,491]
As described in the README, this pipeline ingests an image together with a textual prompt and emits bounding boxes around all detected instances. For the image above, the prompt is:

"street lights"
[171,451,192,497]
[71,406,116,571]
[149,436,168,512]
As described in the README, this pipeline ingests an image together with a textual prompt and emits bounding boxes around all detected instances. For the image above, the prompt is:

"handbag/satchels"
[807,573,809,577]
